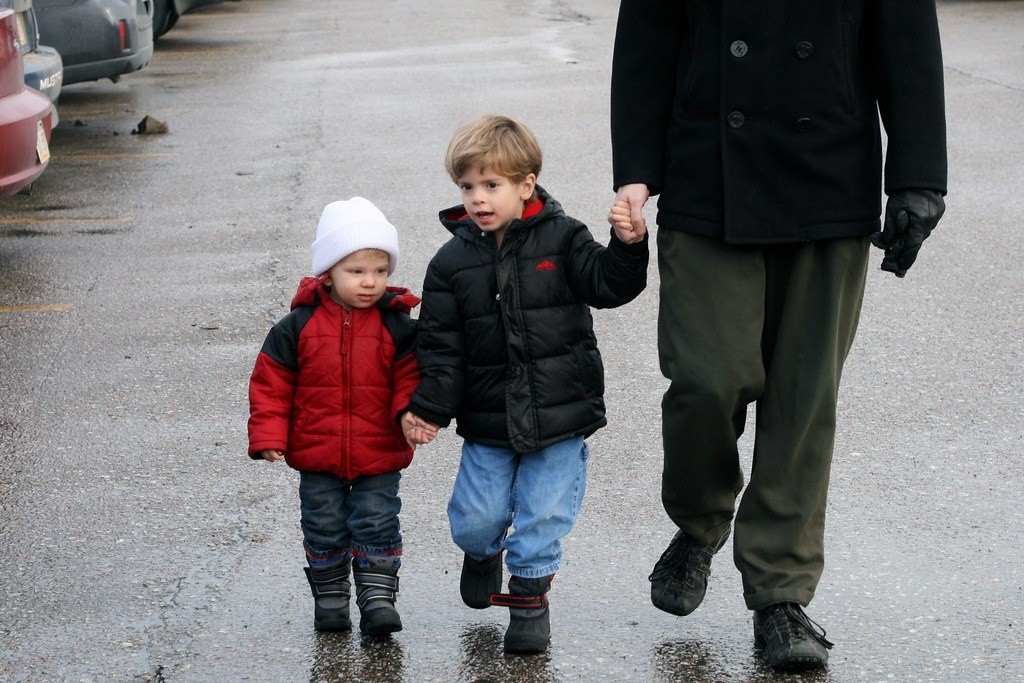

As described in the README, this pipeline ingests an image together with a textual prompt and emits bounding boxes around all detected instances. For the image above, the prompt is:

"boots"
[351,569,404,633]
[459,552,502,610]
[490,572,556,657]
[303,558,353,635]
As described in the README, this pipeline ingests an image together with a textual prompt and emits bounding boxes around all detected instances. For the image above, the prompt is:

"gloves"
[871,189,944,277]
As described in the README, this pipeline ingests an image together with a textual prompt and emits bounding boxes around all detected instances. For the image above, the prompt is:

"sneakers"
[753,602,834,673]
[648,527,731,617]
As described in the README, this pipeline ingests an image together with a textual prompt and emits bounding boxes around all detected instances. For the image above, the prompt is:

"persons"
[607,0,948,663]
[406,114,649,655]
[247,197,437,632]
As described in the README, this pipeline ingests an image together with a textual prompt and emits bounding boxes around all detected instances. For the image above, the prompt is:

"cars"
[0,0,63,112]
[31,0,157,86]
[1,7,54,201]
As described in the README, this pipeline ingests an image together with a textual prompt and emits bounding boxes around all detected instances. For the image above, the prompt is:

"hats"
[311,196,398,277]
